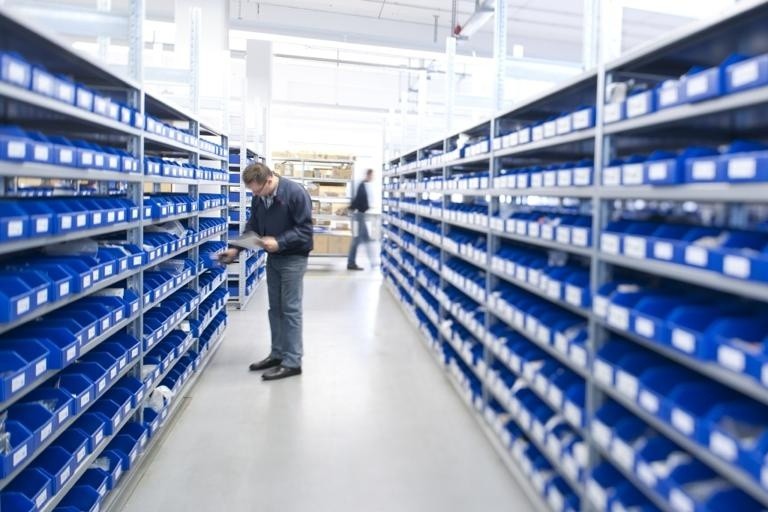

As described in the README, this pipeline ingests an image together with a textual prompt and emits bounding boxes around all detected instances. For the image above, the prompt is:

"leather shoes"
[262,363,301,380]
[249,354,283,371]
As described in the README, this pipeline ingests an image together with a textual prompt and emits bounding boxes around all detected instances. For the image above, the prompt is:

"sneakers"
[347,264,364,271]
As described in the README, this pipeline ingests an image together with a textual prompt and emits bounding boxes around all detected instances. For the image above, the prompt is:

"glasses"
[249,179,268,195]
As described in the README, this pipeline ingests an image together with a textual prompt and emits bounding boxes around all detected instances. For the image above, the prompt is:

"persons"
[344,166,378,270]
[209,162,313,381]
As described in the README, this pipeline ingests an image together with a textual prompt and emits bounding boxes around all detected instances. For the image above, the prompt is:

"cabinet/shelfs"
[381,0,768,511]
[228,146,266,312]
[0,13,145,512]
[278,158,357,258]
[146,94,228,437]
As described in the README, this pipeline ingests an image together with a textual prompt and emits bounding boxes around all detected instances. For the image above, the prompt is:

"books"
[225,232,268,252]
